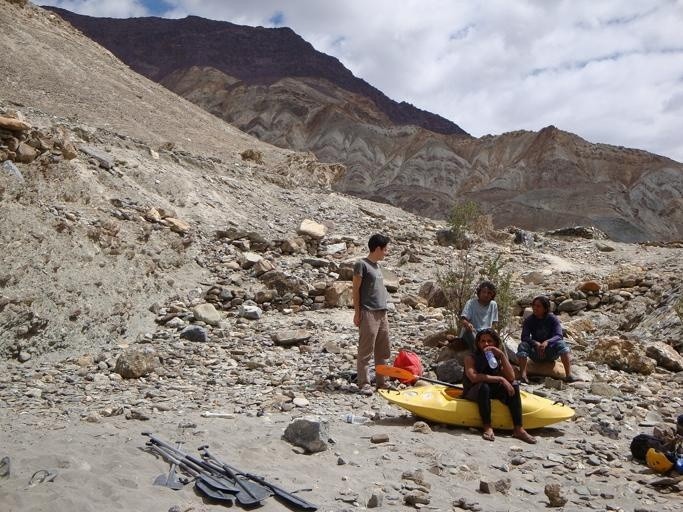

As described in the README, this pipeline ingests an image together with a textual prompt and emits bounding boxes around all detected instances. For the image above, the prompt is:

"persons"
[514,296,578,386]
[351,232,397,396]
[458,280,498,353]
[461,327,540,444]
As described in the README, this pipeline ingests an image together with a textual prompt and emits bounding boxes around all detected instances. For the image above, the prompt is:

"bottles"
[485,350,498,369]
[342,414,368,425]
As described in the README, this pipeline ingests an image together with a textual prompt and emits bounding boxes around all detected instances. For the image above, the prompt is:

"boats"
[378,384,575,430]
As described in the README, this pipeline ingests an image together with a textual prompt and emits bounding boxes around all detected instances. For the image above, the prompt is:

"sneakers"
[358,381,399,395]
[566,375,578,383]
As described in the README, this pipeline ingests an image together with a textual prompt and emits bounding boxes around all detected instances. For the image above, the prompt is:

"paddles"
[375,364,464,390]
[141,431,319,510]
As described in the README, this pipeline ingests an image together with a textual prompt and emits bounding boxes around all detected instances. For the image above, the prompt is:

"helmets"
[646,447,674,474]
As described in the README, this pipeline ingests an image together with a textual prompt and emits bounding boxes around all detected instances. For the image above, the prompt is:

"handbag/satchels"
[393,349,423,385]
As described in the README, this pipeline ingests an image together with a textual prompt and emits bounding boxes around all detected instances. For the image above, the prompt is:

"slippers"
[481,429,496,443]
[512,430,538,445]
